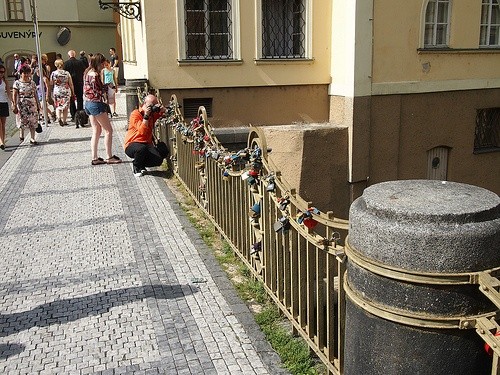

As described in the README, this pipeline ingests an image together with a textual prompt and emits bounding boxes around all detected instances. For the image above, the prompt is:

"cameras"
[149,105,160,113]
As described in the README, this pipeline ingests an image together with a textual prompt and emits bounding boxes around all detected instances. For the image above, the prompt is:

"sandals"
[92,157,107,165]
[107,155,122,164]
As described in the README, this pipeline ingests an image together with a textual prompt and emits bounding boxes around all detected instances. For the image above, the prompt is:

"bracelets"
[143,115,149,120]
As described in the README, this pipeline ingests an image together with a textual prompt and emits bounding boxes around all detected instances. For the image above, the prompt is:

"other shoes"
[141,167,148,173]
[30,140,39,146]
[0,143,5,149]
[133,164,142,177]
[113,112,118,117]
[19,137,24,141]
[58,119,64,127]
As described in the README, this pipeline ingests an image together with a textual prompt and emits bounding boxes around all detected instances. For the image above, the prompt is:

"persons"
[101,59,118,117]
[63,49,86,121]
[82,53,122,165]
[49,59,75,126]
[124,94,170,176]
[0,51,93,150]
[12,65,41,145]
[109,47,119,85]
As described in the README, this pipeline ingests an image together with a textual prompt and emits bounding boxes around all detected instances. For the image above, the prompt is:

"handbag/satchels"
[156,141,169,158]
[35,109,42,133]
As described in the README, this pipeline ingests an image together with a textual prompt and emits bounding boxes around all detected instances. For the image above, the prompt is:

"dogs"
[74,110,90,129]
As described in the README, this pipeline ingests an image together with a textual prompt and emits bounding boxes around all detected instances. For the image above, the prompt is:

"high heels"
[51,112,56,122]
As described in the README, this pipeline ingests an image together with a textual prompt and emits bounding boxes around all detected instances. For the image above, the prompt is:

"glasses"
[0,71,5,73]
